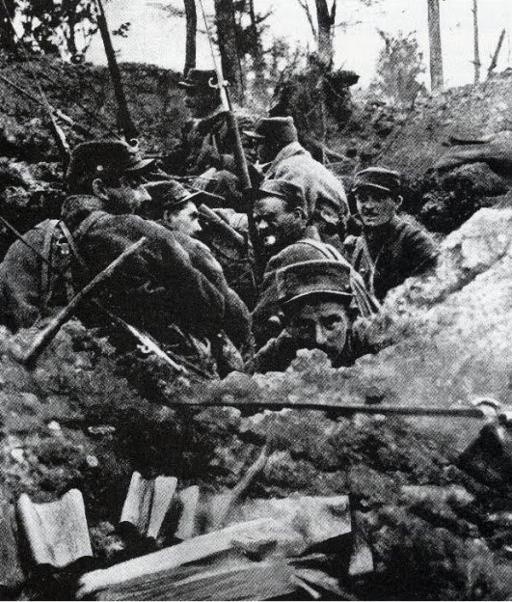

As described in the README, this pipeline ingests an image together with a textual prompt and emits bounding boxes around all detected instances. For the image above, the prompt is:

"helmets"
[262,239,356,304]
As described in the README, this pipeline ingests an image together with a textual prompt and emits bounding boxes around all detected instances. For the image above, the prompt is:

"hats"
[244,115,299,142]
[177,67,218,90]
[73,140,156,178]
[243,181,308,214]
[352,166,401,195]
[140,182,203,218]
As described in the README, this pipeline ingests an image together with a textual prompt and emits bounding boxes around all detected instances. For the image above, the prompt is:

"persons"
[164,66,234,173]
